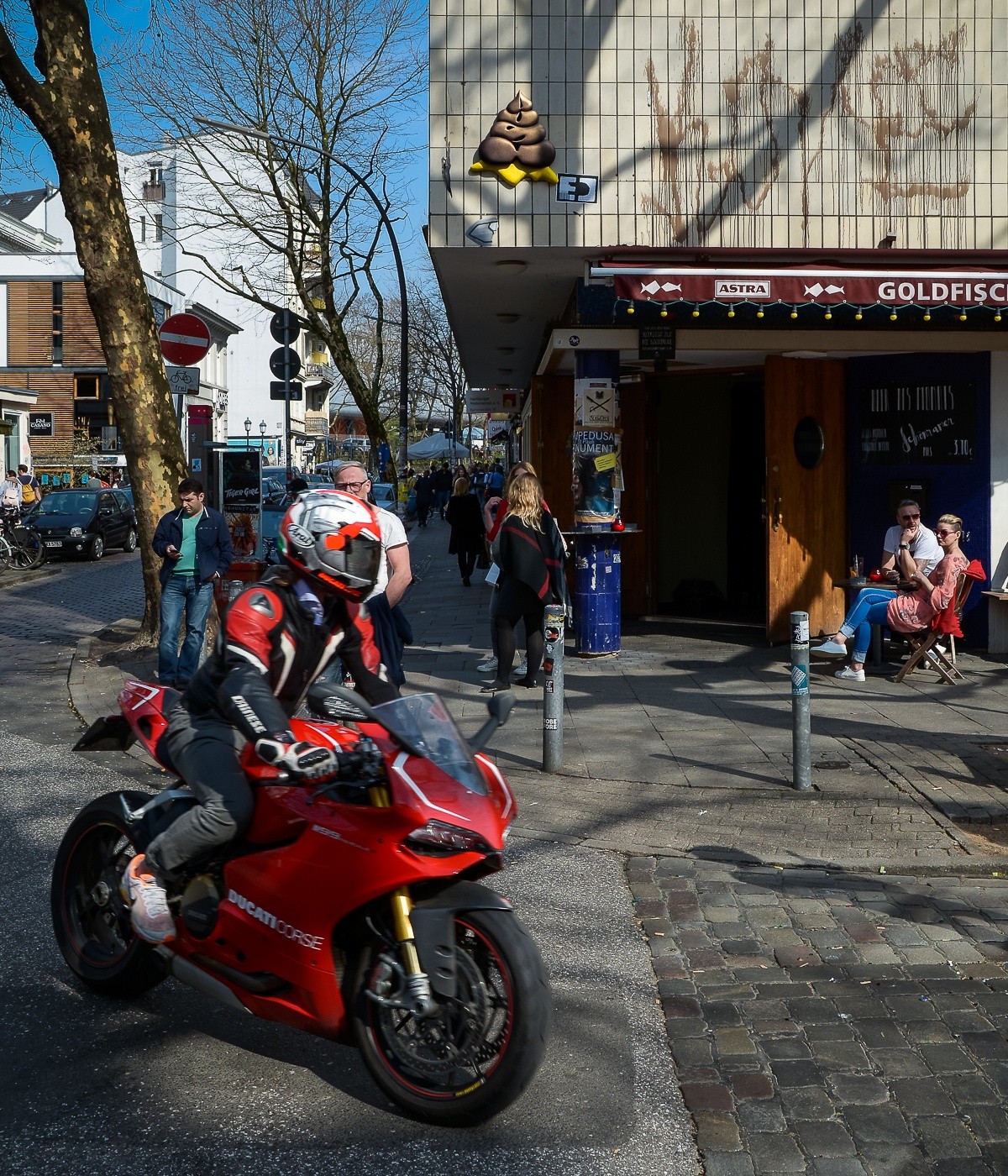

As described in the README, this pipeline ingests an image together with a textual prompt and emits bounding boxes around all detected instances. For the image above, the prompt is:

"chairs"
[887,559,981,684]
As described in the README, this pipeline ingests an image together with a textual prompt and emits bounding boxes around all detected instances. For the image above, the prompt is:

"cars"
[261,464,337,507]
[18,487,139,561]
[371,484,397,514]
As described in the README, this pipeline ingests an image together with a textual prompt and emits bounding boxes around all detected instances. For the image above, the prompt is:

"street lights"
[259,419,267,456]
[244,417,252,452]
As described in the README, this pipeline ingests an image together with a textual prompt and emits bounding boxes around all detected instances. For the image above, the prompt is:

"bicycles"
[0,504,47,572]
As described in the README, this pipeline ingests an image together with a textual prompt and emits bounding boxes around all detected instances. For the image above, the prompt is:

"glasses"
[335,480,368,492]
[933,528,958,538]
[901,514,919,521]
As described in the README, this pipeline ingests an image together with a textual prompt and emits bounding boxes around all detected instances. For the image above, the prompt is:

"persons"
[406,458,564,692]
[881,499,945,582]
[332,461,412,609]
[152,477,232,692]
[260,445,276,466]
[810,514,969,682]
[0,464,127,524]
[121,489,431,943]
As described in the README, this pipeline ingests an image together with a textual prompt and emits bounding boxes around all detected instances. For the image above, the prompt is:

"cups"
[853,557,864,578]
[612,519,625,531]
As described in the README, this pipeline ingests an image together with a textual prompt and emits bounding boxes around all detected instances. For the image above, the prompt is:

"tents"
[408,430,469,460]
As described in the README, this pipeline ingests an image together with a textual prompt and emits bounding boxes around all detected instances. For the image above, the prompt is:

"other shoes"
[418,523,421,527]
[423,523,426,526]
[924,643,947,669]
[481,685,511,692]
[516,678,536,688]
[463,578,471,587]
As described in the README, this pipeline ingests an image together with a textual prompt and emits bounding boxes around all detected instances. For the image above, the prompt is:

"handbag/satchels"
[475,535,490,570]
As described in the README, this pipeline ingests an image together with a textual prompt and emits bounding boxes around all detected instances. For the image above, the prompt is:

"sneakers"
[809,638,847,658]
[513,657,528,674]
[835,665,866,682]
[477,658,500,671]
[119,852,176,943]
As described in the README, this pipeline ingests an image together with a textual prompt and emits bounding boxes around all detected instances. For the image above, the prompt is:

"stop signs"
[157,314,212,367]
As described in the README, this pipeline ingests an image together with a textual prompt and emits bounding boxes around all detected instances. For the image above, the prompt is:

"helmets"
[275,490,382,603]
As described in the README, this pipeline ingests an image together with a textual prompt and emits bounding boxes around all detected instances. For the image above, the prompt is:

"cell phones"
[170,550,184,557]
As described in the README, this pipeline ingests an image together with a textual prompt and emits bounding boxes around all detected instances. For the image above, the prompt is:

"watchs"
[899,542,910,550]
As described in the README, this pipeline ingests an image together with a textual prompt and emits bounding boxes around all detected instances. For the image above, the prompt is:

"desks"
[852,581,917,673]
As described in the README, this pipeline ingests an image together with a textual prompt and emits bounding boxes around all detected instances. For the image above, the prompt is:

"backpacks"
[3,483,19,507]
[16,476,36,504]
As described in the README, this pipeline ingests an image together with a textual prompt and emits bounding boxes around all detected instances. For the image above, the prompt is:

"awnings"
[590,261,1007,307]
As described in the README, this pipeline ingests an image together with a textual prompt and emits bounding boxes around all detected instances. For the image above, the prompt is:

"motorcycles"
[46,676,550,1131]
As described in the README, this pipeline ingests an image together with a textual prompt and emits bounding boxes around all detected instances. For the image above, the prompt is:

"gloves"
[255,728,339,787]
